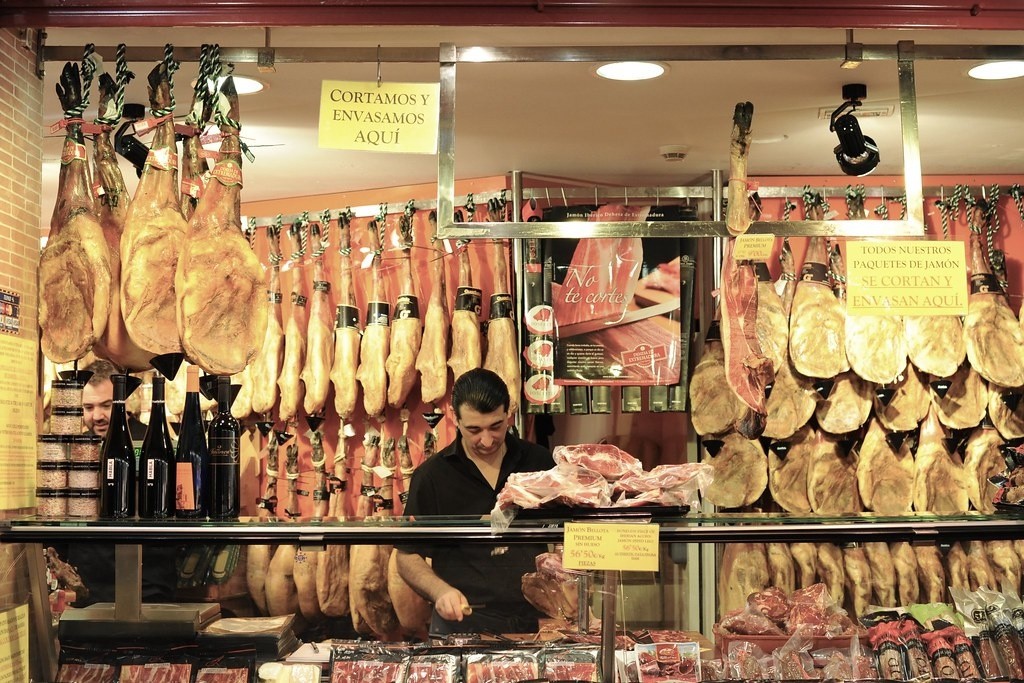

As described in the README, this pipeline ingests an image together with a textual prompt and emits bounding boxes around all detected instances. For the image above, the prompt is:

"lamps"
[829,84,880,176]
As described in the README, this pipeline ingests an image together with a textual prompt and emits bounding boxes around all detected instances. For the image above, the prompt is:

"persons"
[43,361,187,609]
[390,367,566,639]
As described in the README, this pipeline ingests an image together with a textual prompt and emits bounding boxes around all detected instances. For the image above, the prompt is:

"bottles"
[98,374,137,520]
[174,365,209,519]
[138,370,176,520]
[208,376,240,520]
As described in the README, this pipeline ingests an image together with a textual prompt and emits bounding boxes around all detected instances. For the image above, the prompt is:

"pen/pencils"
[464,603,486,608]
